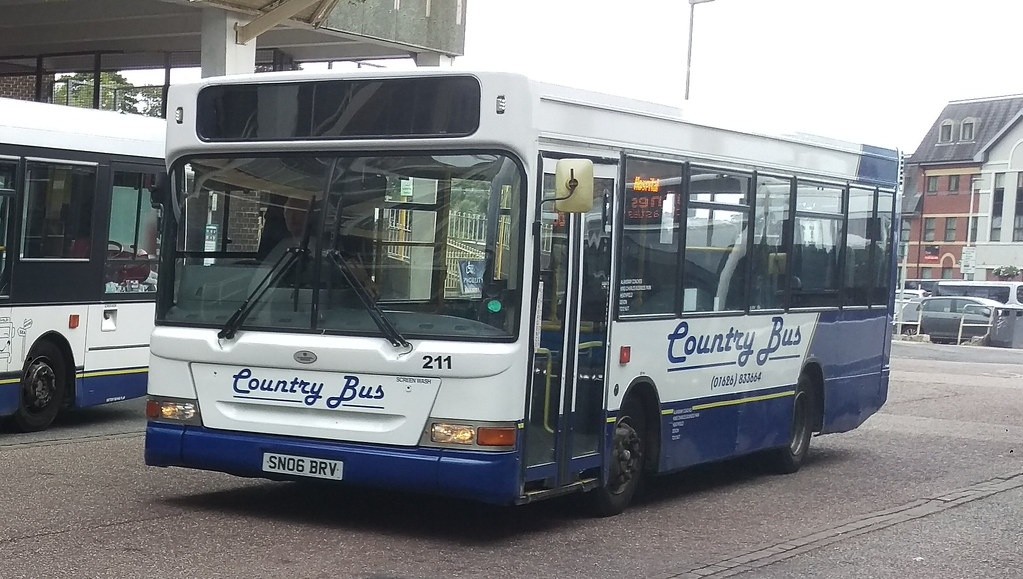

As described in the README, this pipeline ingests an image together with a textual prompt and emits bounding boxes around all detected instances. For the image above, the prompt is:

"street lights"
[684,0,717,101]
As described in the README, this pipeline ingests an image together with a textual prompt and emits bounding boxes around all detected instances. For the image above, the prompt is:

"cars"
[895,289,930,302]
[894,296,1005,343]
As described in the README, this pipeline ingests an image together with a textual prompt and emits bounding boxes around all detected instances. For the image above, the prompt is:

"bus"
[141,71,901,521]
[0,97,220,434]
[898,279,1023,309]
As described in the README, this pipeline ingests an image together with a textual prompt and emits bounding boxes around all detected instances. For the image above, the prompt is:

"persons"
[283,192,382,302]
